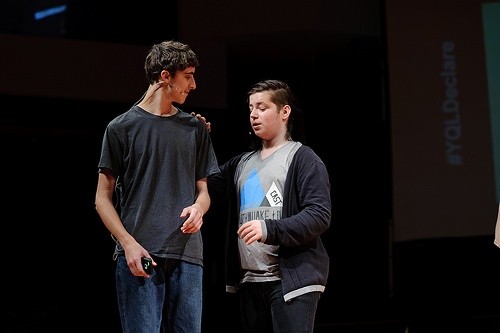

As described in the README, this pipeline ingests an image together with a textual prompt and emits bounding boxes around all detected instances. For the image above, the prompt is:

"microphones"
[249,128,254,135]
[168,82,186,96]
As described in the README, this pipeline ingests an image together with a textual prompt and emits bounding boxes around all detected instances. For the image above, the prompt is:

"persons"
[94,41,224,333]
[189,78,332,333]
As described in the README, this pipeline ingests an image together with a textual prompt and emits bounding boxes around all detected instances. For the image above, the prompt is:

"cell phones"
[137,256,153,277]
